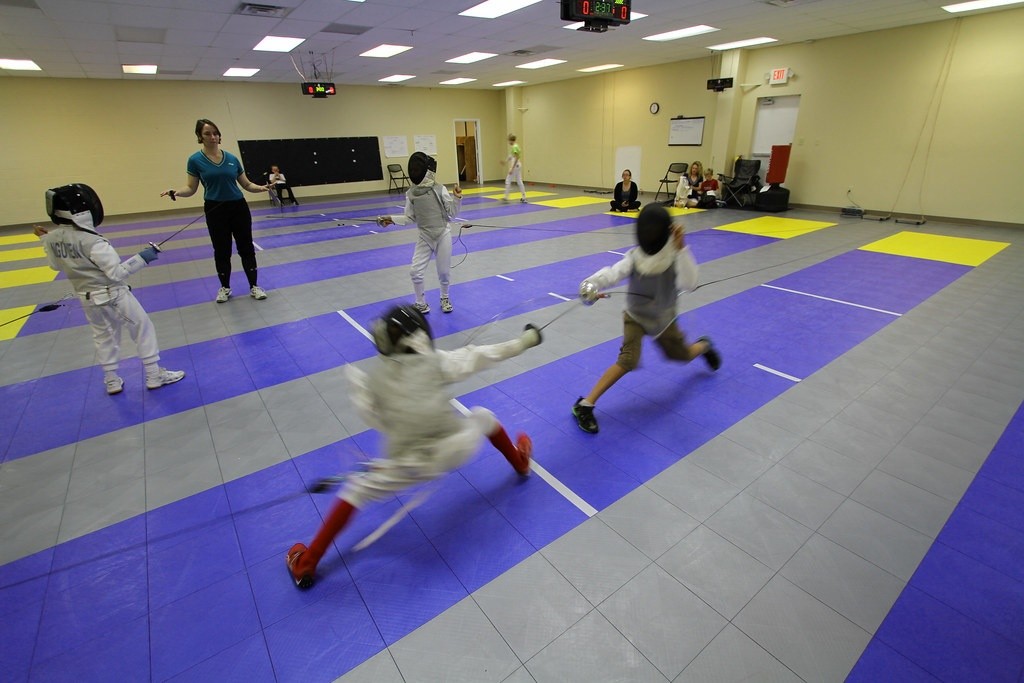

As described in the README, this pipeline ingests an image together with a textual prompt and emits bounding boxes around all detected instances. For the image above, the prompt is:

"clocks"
[650,103,659,114]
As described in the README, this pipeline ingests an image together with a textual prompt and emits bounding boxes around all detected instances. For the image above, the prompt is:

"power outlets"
[847,185,854,193]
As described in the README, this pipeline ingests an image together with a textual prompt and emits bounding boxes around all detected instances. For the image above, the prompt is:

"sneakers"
[517,432,531,477]
[216,286,232,303]
[571,396,598,435]
[103,376,123,394]
[145,367,185,390]
[440,298,454,313]
[410,303,431,314]
[285,543,317,589]
[250,285,267,300]
[696,335,721,371]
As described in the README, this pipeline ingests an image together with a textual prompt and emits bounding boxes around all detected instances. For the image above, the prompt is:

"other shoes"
[520,198,527,203]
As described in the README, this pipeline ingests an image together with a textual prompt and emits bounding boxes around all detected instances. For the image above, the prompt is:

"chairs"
[266,172,290,204]
[654,162,689,199]
[387,164,411,194]
[717,158,762,208]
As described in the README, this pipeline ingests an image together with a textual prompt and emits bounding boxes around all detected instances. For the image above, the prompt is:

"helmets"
[44,183,104,227]
[372,306,433,356]
[408,151,437,185]
[636,202,672,255]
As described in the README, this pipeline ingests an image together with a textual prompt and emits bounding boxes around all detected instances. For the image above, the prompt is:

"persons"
[499,134,528,203]
[572,203,720,434]
[380,151,463,314]
[674,161,719,209]
[610,169,641,212]
[160,119,277,303]
[32,184,185,394]
[270,166,299,206]
[285,304,538,590]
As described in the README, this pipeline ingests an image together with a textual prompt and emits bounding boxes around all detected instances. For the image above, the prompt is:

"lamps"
[763,66,794,84]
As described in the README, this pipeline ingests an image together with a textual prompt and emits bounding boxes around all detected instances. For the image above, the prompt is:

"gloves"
[138,247,159,265]
